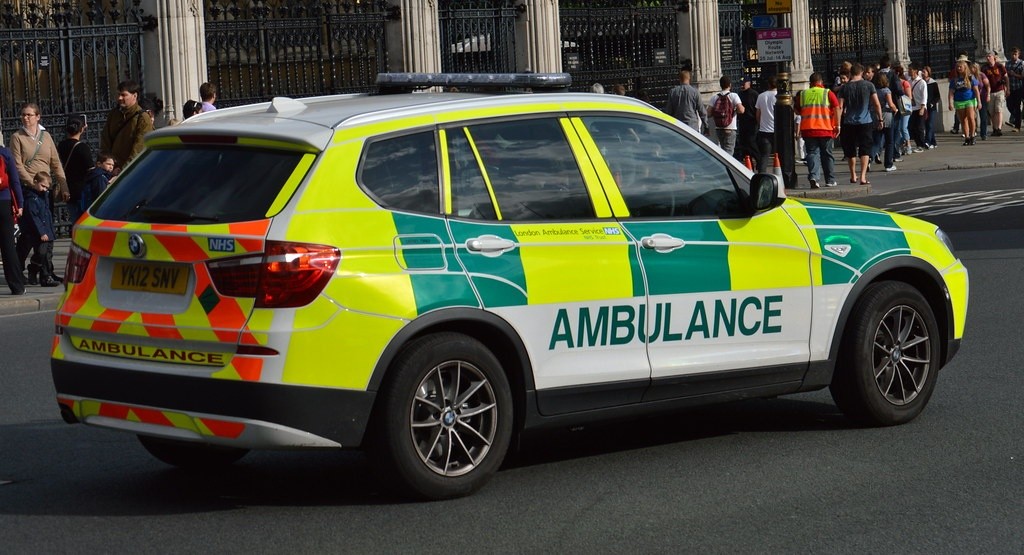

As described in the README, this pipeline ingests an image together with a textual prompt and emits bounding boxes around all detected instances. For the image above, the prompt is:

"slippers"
[860,180,871,185]
[850,176,858,183]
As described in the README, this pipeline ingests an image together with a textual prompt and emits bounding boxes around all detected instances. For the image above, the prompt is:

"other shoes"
[826,181,838,187]
[929,145,938,149]
[40,279,61,287]
[27,270,39,286]
[874,155,882,165]
[912,148,925,153]
[810,178,820,189]
[950,121,1019,146]
[893,158,904,162]
[924,142,930,149]
[50,273,64,283]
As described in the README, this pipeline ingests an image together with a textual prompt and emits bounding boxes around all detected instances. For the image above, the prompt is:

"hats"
[736,75,752,84]
[955,54,972,65]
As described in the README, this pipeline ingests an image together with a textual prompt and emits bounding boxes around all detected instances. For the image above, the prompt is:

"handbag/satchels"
[897,94,913,116]
[872,111,894,129]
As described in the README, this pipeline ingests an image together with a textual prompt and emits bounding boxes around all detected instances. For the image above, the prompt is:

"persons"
[590,48,1024,189]
[0,81,217,295]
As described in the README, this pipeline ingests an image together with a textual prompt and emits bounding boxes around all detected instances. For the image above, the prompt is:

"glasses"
[19,113,37,118]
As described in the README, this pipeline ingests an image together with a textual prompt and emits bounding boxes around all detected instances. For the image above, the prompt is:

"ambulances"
[51,72,972,499]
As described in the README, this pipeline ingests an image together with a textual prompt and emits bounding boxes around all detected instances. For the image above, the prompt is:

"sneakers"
[885,165,897,172]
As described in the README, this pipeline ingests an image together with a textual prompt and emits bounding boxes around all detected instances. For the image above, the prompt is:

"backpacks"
[711,92,737,128]
